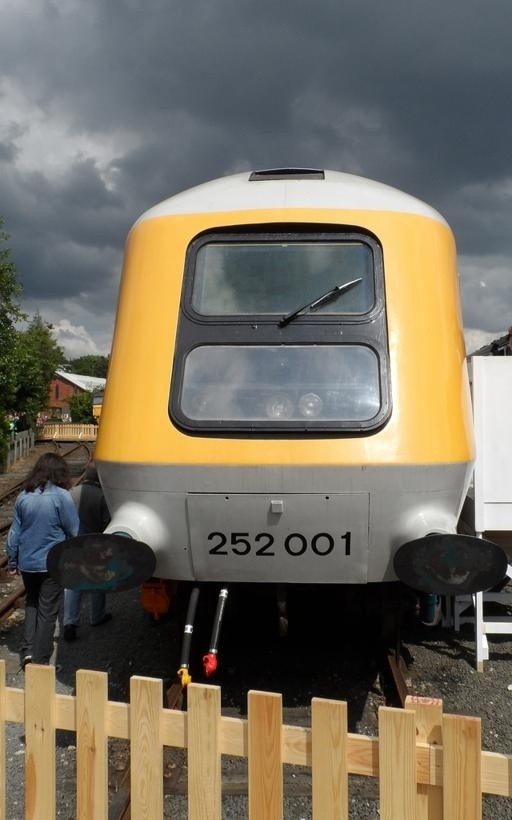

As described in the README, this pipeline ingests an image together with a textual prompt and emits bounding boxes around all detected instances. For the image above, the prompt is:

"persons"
[6,451,88,674]
[61,462,111,641]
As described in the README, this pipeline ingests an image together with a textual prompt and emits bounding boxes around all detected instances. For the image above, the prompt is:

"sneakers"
[90,611,112,626]
[63,625,77,641]
[20,655,33,672]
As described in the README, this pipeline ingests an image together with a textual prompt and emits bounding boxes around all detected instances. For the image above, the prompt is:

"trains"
[46,167,509,685]
